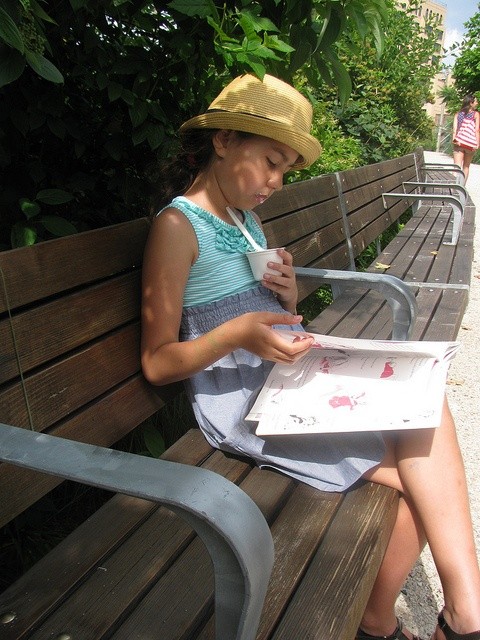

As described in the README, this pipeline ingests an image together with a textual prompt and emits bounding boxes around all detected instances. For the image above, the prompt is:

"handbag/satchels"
[452,117,480,150]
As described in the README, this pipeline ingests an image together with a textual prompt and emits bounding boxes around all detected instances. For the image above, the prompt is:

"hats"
[176,72,321,170]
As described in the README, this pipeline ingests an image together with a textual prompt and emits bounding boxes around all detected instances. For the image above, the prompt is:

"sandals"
[431,606,479,640]
[354,616,424,640]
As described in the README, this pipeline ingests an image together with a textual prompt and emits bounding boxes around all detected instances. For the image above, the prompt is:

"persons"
[142,73,480,640]
[451,97,480,183]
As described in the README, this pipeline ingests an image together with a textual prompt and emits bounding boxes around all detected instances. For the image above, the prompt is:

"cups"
[245,248,283,281]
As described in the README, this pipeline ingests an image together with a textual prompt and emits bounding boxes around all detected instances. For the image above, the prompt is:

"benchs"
[0,213,417,640]
[337,147,476,287]
[153,173,469,342]
